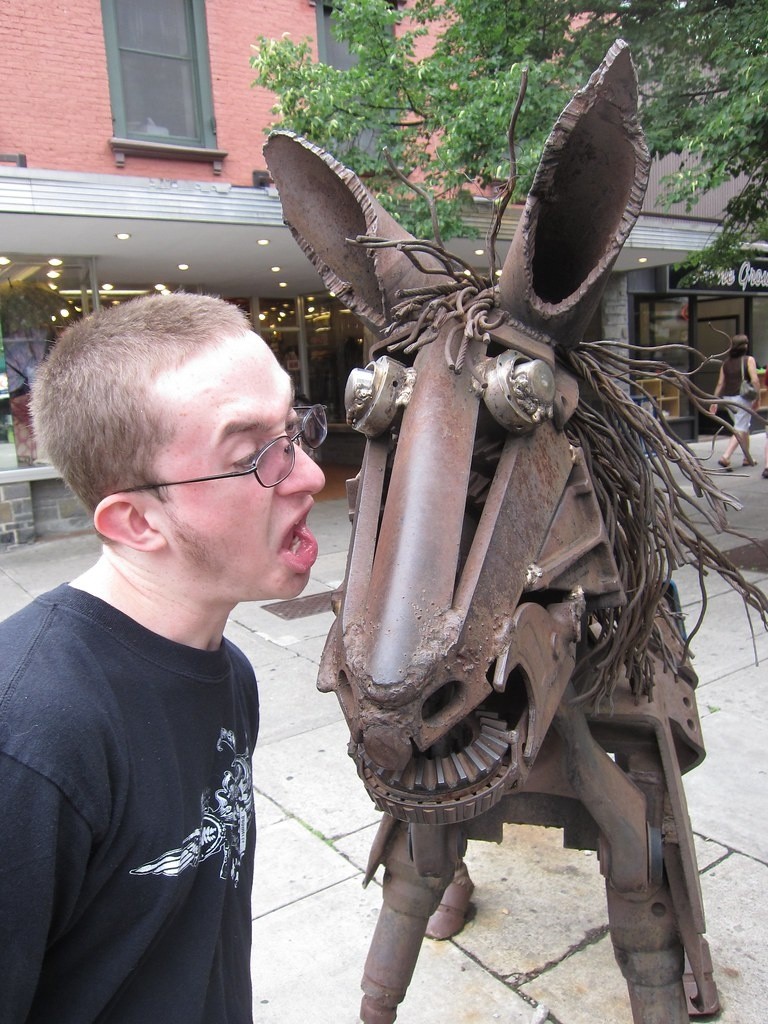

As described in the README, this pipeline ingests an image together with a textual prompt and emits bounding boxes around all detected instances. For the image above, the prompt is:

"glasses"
[110,404,330,494]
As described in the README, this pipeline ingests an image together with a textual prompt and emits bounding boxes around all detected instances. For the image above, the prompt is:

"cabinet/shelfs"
[636,378,680,419]
[752,374,768,410]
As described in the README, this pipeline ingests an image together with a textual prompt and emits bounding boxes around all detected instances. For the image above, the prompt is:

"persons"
[0,291,327,1024]
[762,361,768,479]
[709,335,761,472]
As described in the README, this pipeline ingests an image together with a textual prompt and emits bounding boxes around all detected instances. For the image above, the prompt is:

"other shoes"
[762,469,768,478]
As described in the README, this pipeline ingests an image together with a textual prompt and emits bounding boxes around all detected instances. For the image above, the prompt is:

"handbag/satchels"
[740,356,757,400]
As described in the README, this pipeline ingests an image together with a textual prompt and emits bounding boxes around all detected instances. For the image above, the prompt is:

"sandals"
[742,458,758,466]
[718,456,733,472]
